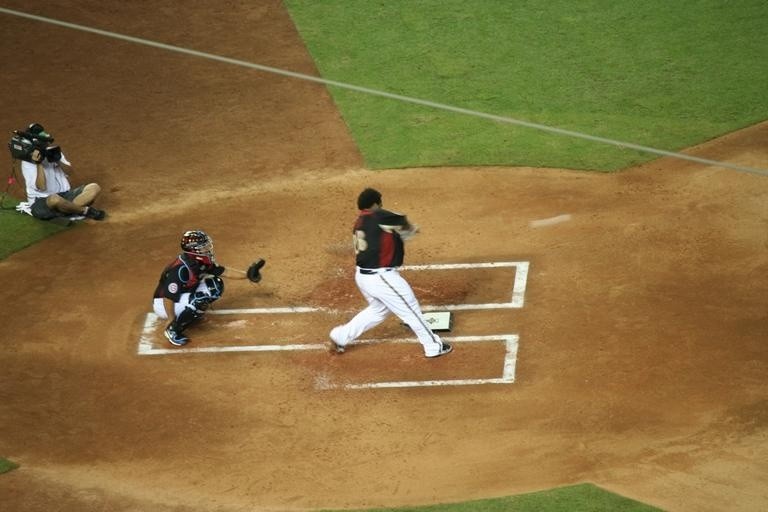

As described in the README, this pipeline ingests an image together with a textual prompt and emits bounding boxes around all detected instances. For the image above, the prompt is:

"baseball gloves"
[246,257,266,283]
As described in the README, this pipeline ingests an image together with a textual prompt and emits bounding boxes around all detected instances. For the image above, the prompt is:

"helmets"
[181,231,212,250]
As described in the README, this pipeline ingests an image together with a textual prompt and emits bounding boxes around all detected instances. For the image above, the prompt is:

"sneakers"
[331,330,345,352]
[164,328,188,346]
[425,345,453,356]
[84,206,104,219]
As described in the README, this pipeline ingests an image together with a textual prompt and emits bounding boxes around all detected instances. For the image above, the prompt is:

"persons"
[152,230,265,346]
[329,188,452,358]
[21,123,105,221]
